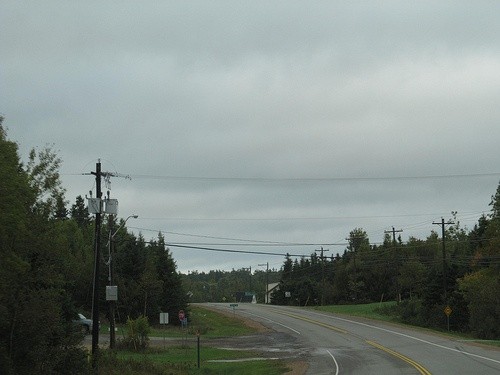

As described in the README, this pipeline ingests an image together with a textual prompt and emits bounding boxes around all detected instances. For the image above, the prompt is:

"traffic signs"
[230,303,239,306]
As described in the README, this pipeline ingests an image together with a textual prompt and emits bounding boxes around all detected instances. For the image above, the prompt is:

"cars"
[72,313,100,334]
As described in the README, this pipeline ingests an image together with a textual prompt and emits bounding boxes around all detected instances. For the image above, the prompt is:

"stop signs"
[179,310,184,320]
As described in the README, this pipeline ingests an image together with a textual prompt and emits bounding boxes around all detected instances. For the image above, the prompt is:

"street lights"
[107,213,138,349]
